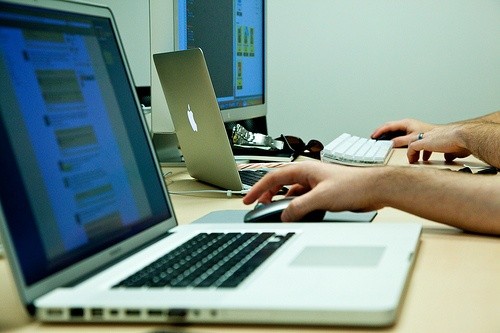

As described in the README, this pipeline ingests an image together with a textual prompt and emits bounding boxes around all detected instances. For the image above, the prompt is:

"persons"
[243,108,500,235]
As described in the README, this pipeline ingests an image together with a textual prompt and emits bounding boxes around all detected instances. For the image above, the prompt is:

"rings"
[418,133,424,140]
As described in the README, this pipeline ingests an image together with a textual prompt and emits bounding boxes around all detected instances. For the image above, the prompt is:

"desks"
[0,146,500,333]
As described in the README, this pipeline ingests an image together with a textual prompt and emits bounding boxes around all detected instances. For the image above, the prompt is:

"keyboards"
[320,133,396,168]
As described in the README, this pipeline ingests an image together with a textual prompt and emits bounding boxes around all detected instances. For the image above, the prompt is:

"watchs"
[232,124,284,150]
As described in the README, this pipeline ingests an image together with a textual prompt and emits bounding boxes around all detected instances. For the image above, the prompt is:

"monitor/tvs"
[171,0,268,124]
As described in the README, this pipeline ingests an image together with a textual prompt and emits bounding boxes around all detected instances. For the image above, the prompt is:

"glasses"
[273,133,324,160]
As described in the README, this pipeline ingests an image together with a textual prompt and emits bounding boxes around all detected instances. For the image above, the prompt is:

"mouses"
[376,129,409,147]
[240,196,327,221]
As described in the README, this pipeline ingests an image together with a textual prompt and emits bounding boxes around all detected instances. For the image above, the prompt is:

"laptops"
[152,47,294,195]
[0,0,423,328]
